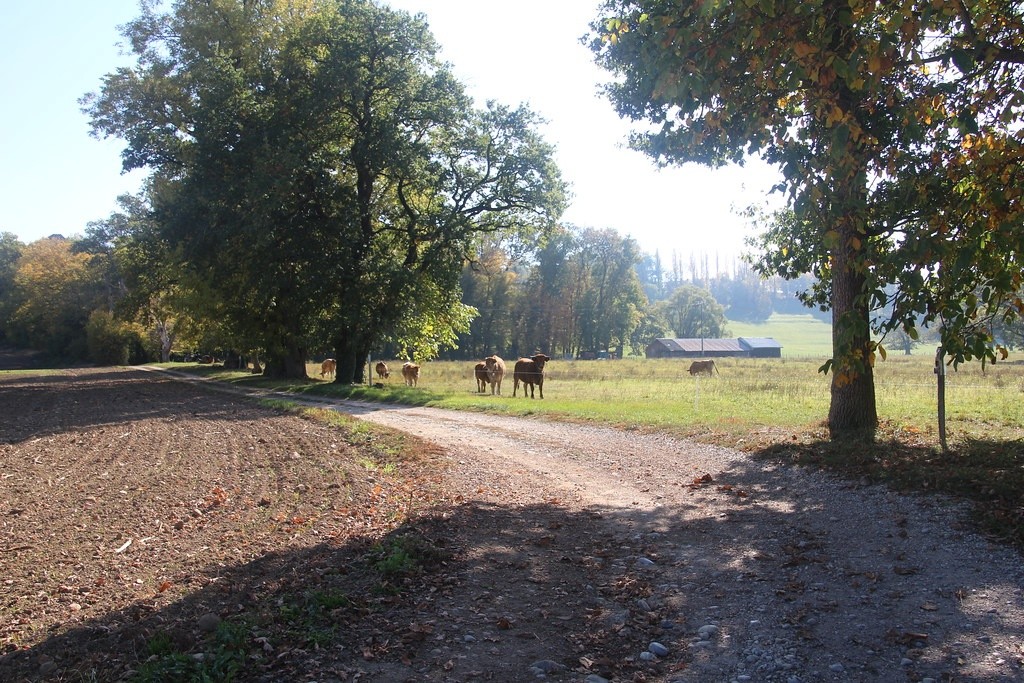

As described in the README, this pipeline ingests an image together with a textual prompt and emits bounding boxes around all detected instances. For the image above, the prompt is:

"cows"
[317,359,337,379]
[686,360,719,376]
[375,362,390,380]
[474,353,550,398]
[401,362,420,387]
